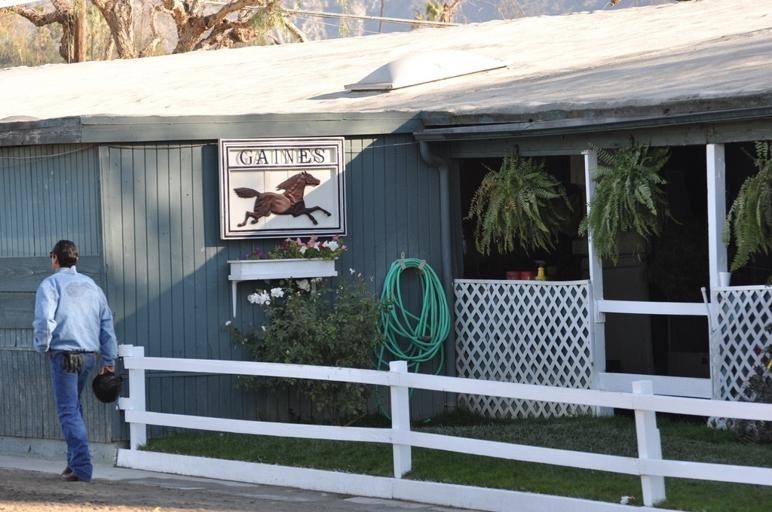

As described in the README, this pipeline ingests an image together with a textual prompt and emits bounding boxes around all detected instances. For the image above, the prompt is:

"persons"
[30,239,118,481]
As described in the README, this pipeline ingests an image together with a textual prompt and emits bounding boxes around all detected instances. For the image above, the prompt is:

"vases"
[226,257,338,275]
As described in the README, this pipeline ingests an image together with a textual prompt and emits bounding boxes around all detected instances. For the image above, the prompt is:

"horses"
[232,170,333,227]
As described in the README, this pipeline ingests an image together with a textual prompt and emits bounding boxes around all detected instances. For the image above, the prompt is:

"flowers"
[242,234,347,261]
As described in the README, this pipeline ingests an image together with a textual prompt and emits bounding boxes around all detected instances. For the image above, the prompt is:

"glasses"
[49,251,55,258]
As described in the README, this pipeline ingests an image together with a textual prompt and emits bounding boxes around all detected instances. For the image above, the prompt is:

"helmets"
[92,372,123,402]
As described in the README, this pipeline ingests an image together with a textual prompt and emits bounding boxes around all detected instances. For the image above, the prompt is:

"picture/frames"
[217,137,348,241]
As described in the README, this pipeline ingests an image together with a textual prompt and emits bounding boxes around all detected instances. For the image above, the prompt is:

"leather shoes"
[59,467,78,481]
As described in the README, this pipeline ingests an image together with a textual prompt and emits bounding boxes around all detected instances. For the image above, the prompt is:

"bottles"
[506,267,547,280]
[719,271,731,287]
